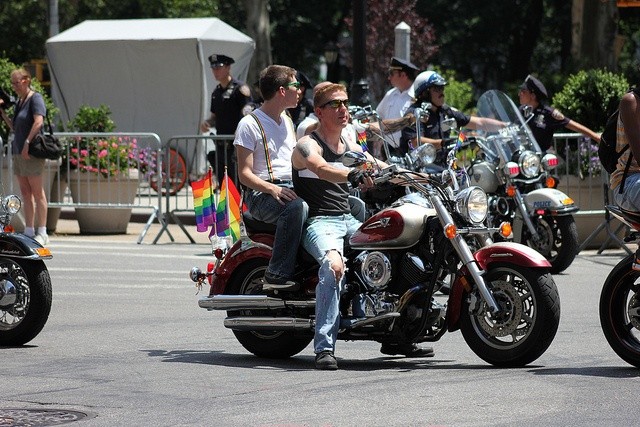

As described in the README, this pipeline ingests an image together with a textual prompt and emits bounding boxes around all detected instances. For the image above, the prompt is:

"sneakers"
[263,276,296,291]
[33,233,49,246]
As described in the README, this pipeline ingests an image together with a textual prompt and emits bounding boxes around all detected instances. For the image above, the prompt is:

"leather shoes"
[380,344,435,357]
[314,351,338,369]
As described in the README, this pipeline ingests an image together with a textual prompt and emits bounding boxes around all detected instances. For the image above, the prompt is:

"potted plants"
[43,130,69,236]
[0,59,62,235]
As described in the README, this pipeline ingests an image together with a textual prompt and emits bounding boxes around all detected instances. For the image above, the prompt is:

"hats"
[519,74,548,96]
[209,55,235,67]
[295,71,315,89]
[384,56,421,73]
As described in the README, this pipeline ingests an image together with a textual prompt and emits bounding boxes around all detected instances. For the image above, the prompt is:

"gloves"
[442,135,465,148]
[429,172,453,187]
[347,169,375,188]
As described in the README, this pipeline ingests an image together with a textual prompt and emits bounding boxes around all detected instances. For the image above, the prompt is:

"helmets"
[414,71,449,96]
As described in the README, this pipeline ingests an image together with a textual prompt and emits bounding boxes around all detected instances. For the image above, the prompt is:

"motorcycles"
[0,187,53,349]
[598,181,640,372]
[189,100,561,368]
[345,102,437,171]
[380,89,579,276]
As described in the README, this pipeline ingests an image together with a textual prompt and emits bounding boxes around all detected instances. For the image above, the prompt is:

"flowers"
[64,134,149,176]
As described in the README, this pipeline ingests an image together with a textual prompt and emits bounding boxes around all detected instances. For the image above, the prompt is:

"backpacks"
[598,89,640,173]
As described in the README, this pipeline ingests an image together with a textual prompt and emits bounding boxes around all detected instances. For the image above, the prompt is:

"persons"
[234,66,308,292]
[373,54,423,156]
[201,51,252,203]
[8,68,49,247]
[405,70,515,185]
[509,69,601,191]
[293,80,436,369]
[609,63,640,218]
[296,83,429,158]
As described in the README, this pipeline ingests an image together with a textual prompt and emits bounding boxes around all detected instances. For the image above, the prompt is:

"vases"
[66,167,140,235]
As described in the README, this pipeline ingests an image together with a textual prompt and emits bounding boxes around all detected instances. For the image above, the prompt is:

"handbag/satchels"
[28,134,64,160]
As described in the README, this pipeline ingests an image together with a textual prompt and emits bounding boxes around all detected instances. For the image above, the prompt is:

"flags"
[208,173,247,249]
[192,167,216,232]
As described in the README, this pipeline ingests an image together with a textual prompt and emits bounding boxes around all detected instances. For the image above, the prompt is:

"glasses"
[519,88,533,94]
[276,81,301,89]
[10,79,28,87]
[429,86,445,92]
[389,70,404,76]
[319,98,352,108]
